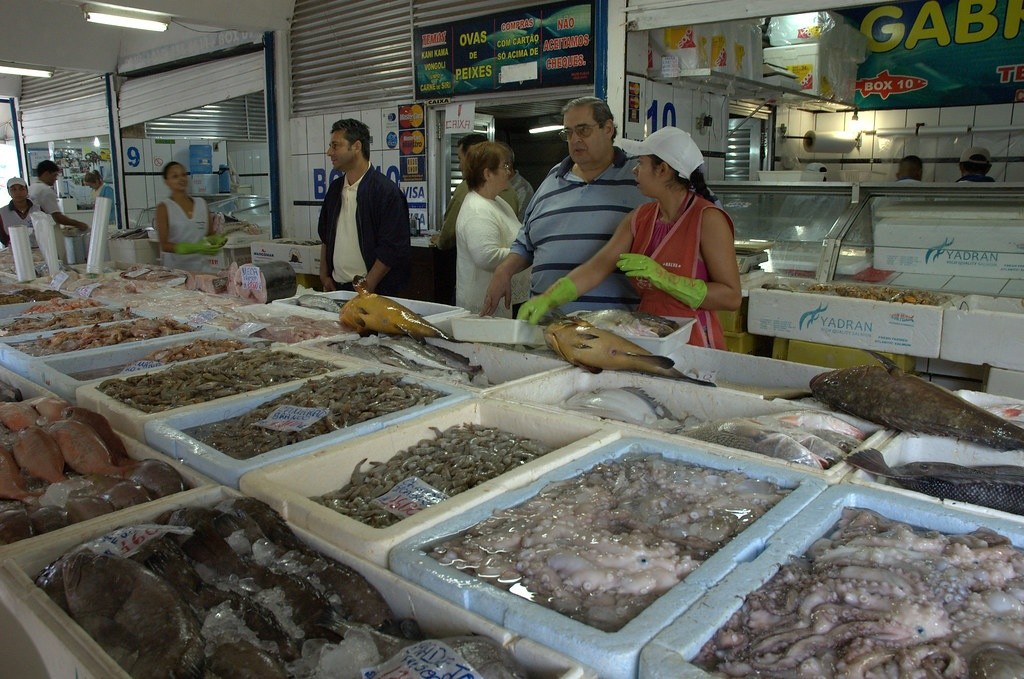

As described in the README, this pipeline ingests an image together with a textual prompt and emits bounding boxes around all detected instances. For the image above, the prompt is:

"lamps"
[0,62,56,78]
[79,3,172,33]
[528,115,564,135]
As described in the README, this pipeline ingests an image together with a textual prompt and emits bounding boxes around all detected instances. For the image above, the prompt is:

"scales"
[734,241,775,274]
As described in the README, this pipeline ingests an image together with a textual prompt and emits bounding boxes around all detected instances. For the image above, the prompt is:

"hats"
[7,176,26,187]
[622,125,705,179]
[960,147,991,164]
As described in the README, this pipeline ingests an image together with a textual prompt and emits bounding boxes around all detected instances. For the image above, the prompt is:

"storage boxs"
[238,183,253,195]
[762,43,856,104]
[0,208,1024,679]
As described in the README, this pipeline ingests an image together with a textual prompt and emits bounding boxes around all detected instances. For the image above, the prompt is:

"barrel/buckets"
[239,183,252,195]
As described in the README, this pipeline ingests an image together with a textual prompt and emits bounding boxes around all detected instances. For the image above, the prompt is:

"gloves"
[177,239,222,257]
[208,231,228,247]
[517,277,578,325]
[616,253,707,312]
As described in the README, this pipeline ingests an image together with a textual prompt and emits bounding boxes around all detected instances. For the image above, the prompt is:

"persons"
[0,177,47,249]
[433,131,535,318]
[154,162,228,273]
[82,169,116,227]
[29,160,88,264]
[480,97,724,325]
[319,118,411,296]
[516,126,742,352]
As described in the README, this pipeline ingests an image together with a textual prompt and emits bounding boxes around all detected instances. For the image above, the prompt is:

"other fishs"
[810,347,1024,451]
[847,446,1024,516]
[30,494,397,678]
[340,274,474,343]
[561,386,864,472]
[1,401,186,544]
[544,308,717,390]
[315,331,483,384]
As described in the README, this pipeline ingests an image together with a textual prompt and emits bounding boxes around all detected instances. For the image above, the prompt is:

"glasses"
[494,165,510,171]
[559,121,603,142]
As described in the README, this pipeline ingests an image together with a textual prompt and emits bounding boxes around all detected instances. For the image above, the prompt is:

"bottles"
[189,145,212,174]
[409,212,426,237]
[458,105,462,119]
[217,164,231,193]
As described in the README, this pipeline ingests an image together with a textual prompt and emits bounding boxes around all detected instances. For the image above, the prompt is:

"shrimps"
[95,347,551,525]
[0,296,450,453]
